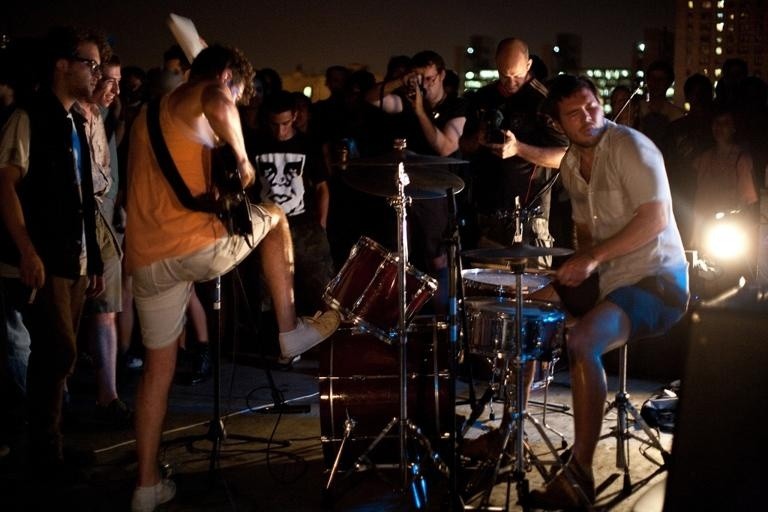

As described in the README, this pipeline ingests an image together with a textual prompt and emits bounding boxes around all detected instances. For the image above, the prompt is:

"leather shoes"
[186,344,213,386]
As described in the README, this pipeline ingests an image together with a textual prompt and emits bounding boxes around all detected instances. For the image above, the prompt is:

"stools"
[551,325,670,495]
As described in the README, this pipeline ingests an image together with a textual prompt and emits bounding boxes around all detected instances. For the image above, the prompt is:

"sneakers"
[523,467,594,509]
[456,427,531,465]
[275,309,341,360]
[93,398,135,423]
[116,349,143,370]
[128,479,177,509]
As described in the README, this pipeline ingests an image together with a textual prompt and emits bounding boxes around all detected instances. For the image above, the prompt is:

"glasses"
[421,74,440,86]
[76,56,100,75]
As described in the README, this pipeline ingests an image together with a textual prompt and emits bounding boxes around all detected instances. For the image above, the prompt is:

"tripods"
[469,259,580,512]
[341,189,451,511]
[157,282,296,500]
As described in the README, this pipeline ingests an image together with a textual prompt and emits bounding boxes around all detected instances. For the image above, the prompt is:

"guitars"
[209,137,253,236]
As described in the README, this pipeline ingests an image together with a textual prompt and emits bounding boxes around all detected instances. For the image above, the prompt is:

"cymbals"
[458,243,574,257]
[356,168,465,201]
[344,147,469,167]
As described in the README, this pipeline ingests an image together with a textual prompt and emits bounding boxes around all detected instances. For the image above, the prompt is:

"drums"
[463,267,551,305]
[320,238,438,352]
[459,295,565,363]
[319,318,452,486]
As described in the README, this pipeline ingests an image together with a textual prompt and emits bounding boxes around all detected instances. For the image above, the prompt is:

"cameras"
[483,108,506,143]
[408,73,423,96]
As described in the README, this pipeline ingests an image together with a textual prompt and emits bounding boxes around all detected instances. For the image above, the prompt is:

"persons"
[0,30,768,512]
